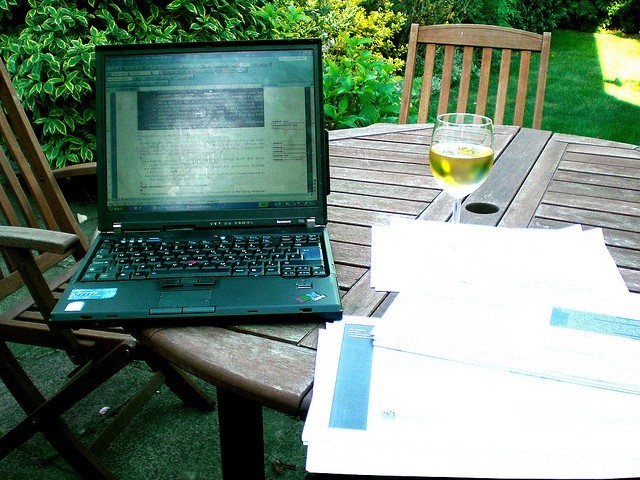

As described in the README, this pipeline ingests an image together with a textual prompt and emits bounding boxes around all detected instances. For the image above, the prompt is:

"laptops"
[48,39,343,328]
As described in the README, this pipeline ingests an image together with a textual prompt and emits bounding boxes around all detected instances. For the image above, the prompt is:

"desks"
[90,123,640,480]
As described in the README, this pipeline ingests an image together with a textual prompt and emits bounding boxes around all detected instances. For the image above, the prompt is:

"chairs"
[397,22,552,131]
[0,58,215,479]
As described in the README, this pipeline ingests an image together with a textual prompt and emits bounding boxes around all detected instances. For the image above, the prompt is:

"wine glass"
[429,113,494,225]
